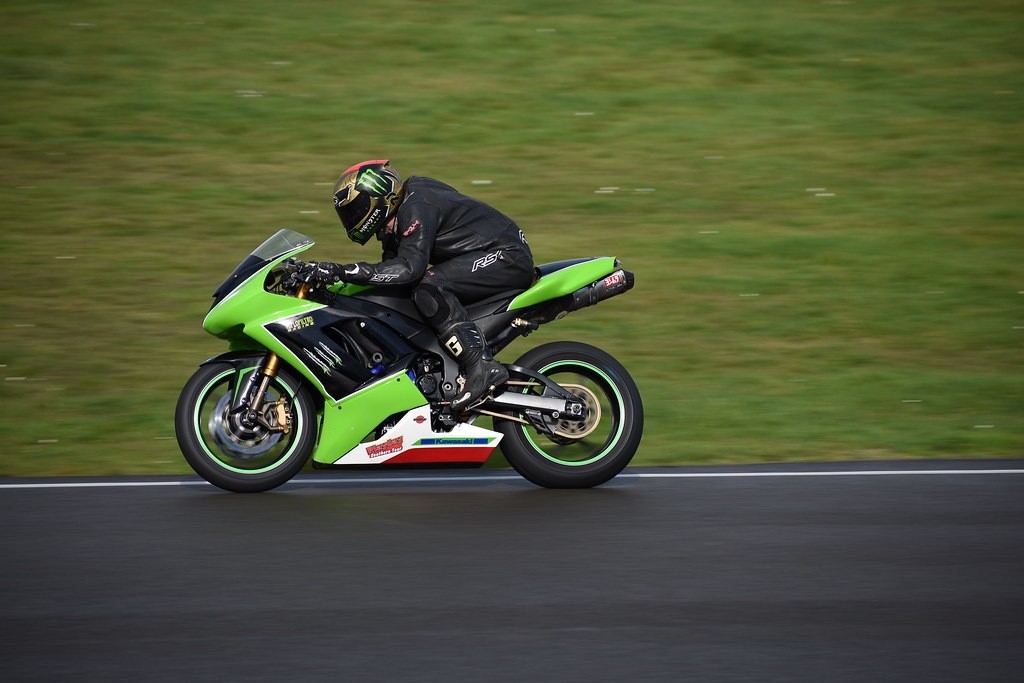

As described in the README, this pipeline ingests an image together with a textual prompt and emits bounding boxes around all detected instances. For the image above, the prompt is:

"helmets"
[333,158,404,244]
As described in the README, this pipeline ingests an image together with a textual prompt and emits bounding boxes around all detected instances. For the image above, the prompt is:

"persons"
[281,160,535,413]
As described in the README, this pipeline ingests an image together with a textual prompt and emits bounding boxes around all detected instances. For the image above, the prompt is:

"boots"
[439,320,509,409]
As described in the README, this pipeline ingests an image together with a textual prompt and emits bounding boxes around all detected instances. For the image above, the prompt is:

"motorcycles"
[174,227,646,490]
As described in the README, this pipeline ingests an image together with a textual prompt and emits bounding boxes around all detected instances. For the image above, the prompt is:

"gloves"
[320,260,374,285]
